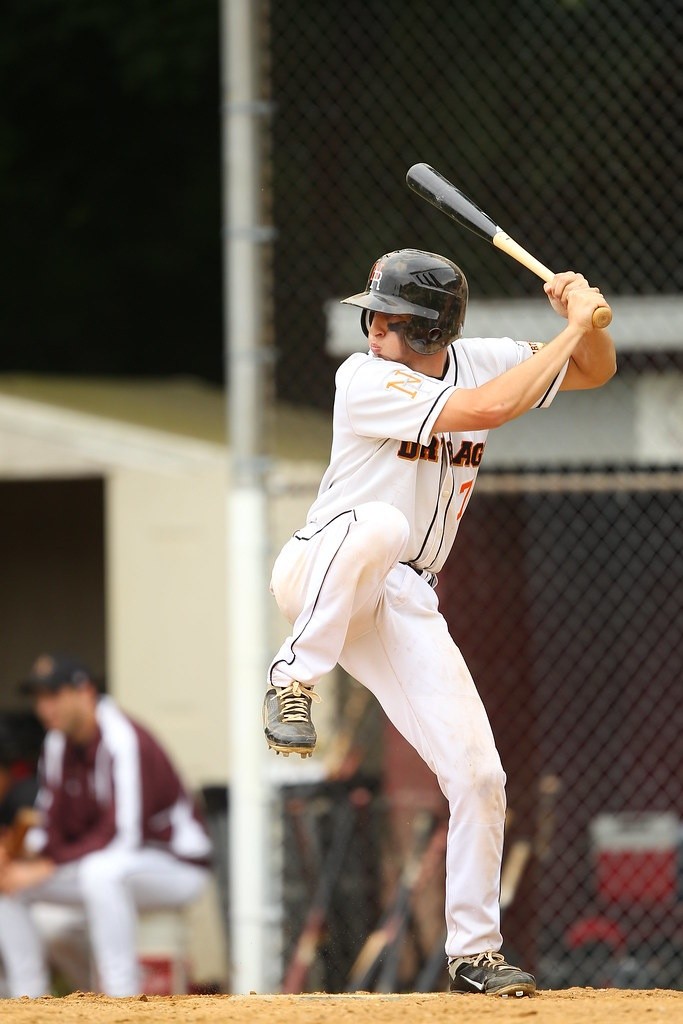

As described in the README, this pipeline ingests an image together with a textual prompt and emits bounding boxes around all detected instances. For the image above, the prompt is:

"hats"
[16,644,101,692]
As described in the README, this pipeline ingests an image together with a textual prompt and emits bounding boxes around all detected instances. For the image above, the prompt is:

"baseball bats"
[405,162,613,329]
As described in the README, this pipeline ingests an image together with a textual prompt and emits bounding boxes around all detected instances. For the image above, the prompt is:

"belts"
[399,559,437,588]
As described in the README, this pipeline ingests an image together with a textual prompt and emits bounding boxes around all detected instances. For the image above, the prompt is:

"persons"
[261,249,618,1002]
[0,653,218,1000]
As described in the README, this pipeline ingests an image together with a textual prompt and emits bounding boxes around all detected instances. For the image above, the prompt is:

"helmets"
[342,248,470,355]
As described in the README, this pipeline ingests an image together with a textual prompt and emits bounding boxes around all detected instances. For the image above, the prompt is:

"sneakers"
[261,679,319,759]
[446,949,536,999]
